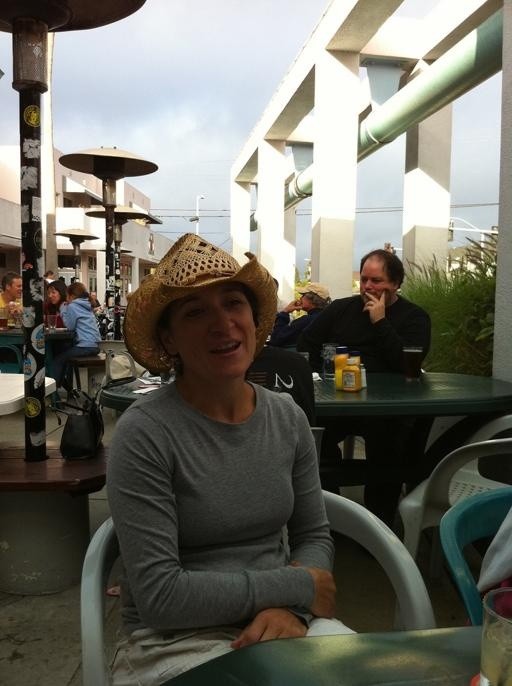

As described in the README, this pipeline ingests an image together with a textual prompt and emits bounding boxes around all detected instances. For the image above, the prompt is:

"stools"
[72,351,107,405]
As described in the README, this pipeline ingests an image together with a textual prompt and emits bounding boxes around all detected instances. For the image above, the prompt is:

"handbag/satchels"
[60,400,104,460]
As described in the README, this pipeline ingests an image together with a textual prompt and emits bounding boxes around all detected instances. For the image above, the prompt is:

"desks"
[0,373,56,416]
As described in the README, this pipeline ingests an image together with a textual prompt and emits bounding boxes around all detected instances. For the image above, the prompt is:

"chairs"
[393,414,512,632]
[439,485,512,626]
[80,488,436,686]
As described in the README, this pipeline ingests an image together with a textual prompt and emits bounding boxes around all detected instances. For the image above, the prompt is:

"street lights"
[194,192,207,234]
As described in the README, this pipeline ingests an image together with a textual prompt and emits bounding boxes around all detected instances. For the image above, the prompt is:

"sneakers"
[67,388,80,402]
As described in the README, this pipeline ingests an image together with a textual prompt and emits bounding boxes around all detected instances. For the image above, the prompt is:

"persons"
[0,270,103,408]
[296,248,431,559]
[270,281,333,351]
[106,233,359,686]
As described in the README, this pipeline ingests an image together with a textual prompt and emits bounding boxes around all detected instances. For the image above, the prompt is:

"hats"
[122,233,278,374]
[295,283,330,301]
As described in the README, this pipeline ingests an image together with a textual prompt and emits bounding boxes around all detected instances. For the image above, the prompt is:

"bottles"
[334,347,367,392]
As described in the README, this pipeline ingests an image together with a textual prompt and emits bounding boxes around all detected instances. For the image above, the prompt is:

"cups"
[0,307,23,331]
[480,586,512,686]
[43,314,57,330]
[323,343,337,380]
